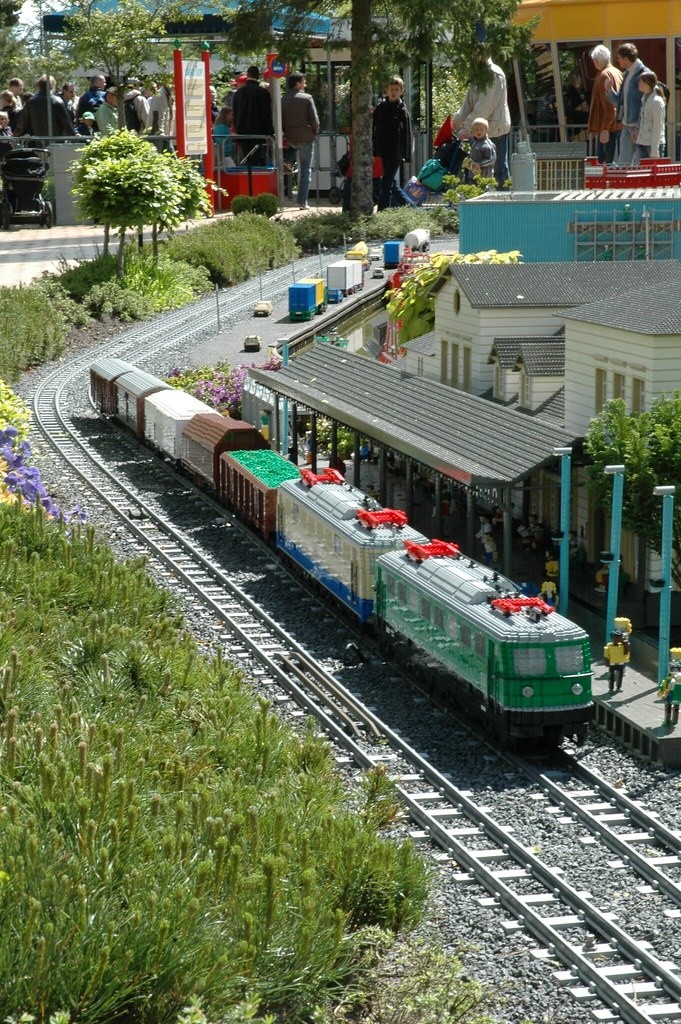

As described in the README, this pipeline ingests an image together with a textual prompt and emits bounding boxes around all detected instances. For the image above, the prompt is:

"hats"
[106,86,118,96]
[83,112,96,121]
[231,75,247,87]
[127,77,140,88]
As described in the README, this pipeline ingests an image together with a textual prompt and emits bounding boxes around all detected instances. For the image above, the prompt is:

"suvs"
[243,335,262,353]
[372,267,385,279]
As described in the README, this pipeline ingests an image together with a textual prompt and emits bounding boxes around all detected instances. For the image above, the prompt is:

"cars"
[326,288,344,304]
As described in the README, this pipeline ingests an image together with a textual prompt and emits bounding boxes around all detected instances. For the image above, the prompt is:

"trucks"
[404,229,431,253]
[384,241,405,269]
[253,301,272,317]
[370,248,383,262]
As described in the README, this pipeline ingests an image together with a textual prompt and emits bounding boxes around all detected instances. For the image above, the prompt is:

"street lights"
[603,464,624,646]
[277,337,291,457]
[653,484,675,687]
[553,448,572,618]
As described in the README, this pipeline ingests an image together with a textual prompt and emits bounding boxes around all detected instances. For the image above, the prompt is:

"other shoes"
[300,205,310,210]
[342,209,350,214]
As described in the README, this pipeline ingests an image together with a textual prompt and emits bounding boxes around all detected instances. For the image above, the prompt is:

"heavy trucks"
[327,260,364,296]
[288,279,328,322]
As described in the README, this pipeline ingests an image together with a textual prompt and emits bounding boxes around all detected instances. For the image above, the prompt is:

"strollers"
[328,151,379,206]
[0,147,53,230]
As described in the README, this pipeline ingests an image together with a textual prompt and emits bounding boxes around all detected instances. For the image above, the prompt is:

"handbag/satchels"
[337,153,349,176]
[418,159,451,193]
[403,174,427,206]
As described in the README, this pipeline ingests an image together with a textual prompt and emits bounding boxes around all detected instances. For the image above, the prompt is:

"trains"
[88,355,594,760]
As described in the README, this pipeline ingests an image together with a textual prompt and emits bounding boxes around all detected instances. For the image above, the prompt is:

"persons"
[281,75,319,210]
[566,72,591,133]
[453,46,511,188]
[0,73,178,210]
[211,67,273,168]
[587,44,624,162]
[372,78,413,210]
[634,72,665,163]
[341,102,382,213]
[260,409,272,440]
[658,647,681,726]
[305,431,454,535]
[605,43,652,166]
[460,118,496,178]
[603,617,632,694]
[477,507,623,610]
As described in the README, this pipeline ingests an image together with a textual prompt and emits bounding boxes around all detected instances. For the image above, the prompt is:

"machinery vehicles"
[344,240,372,271]
